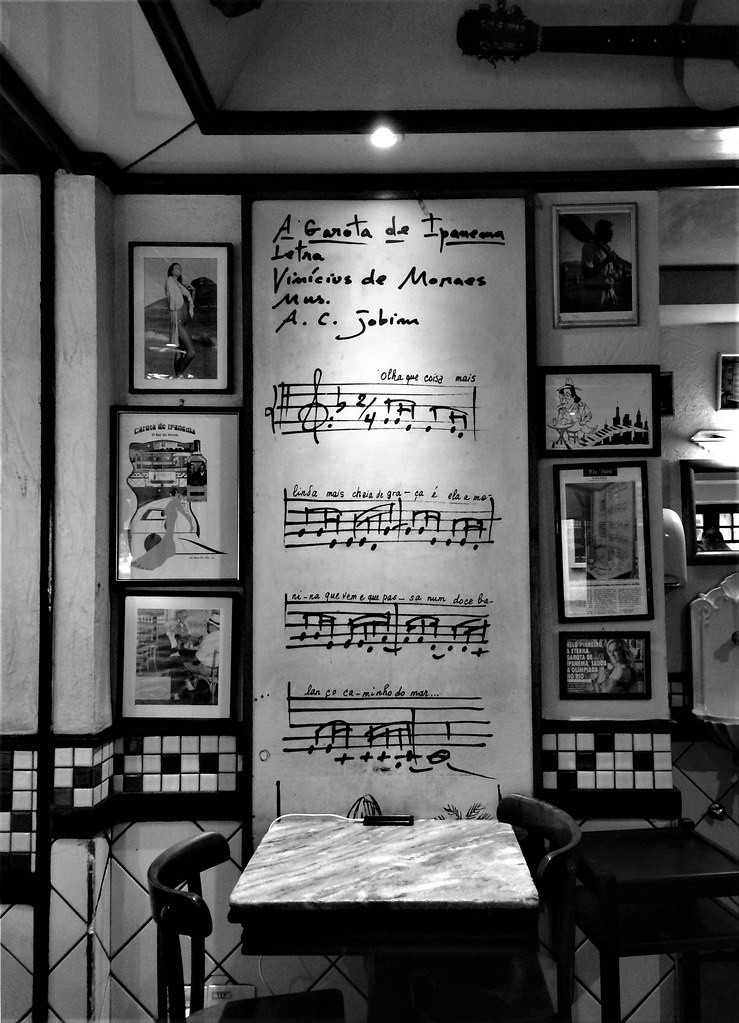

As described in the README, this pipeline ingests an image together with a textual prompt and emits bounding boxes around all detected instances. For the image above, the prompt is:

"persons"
[164,263,196,377]
[583,220,620,312]
[154,610,220,704]
[595,640,640,694]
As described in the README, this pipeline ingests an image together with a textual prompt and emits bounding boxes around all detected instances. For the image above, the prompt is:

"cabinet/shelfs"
[550,827,739,1023]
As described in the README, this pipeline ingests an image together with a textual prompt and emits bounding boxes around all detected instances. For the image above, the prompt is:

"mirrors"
[138,0,739,137]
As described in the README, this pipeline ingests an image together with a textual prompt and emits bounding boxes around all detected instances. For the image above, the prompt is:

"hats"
[204,614,219,630]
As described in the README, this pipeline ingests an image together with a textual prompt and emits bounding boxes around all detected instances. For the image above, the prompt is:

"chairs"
[146,828,344,1023]
[394,793,582,1023]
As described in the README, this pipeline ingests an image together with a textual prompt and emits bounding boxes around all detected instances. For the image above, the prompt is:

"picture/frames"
[679,458,739,566]
[557,628,652,699]
[716,352,739,414]
[111,590,243,727]
[127,240,236,392]
[553,205,640,331]
[108,404,250,585]
[552,460,653,623]
[532,364,662,458]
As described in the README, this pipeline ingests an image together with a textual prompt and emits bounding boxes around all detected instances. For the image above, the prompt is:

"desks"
[226,814,539,1023]
